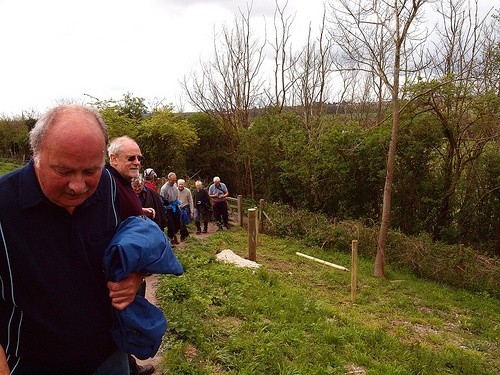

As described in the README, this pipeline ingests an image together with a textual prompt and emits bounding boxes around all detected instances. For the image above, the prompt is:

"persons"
[131,168,193,247]
[208,177,230,231]
[192,181,209,234]
[0,106,144,375]
[108,135,155,375]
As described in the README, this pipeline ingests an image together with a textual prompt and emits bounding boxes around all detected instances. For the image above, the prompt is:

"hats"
[143,168,157,177]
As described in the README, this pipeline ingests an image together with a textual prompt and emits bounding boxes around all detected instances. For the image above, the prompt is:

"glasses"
[125,156,144,161]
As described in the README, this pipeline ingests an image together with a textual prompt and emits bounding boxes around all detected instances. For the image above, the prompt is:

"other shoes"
[170,241,180,248]
[196,229,207,234]
[216,229,223,232]
